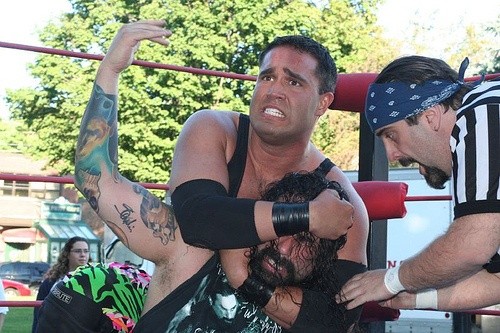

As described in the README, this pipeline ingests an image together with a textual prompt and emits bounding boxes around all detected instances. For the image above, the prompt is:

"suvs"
[0,261,49,294]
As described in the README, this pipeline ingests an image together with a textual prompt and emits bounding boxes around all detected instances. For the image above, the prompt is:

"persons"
[177,282,236,333]
[335,57,500,333]
[0,279,10,333]
[74,22,348,333]
[167,36,368,333]
[35,262,152,333]
[32,237,90,333]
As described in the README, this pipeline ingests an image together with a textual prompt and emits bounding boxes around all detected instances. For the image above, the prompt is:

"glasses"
[69,249,90,255]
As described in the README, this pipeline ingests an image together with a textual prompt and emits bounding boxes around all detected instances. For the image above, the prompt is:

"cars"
[1,280,33,297]
